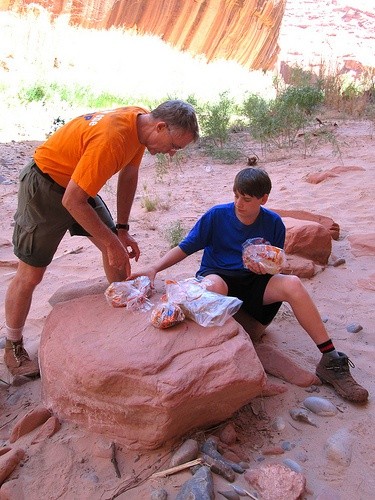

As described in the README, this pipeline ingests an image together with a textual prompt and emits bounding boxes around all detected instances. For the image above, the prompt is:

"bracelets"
[115,224,130,232]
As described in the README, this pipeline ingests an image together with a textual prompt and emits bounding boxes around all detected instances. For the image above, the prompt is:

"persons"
[2,100,200,379]
[124,167,370,402]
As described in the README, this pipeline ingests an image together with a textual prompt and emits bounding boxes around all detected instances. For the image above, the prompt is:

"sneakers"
[3,335,40,377]
[315,351,369,401]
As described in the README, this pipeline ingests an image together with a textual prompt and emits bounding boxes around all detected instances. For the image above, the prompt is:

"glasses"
[166,123,179,152]
